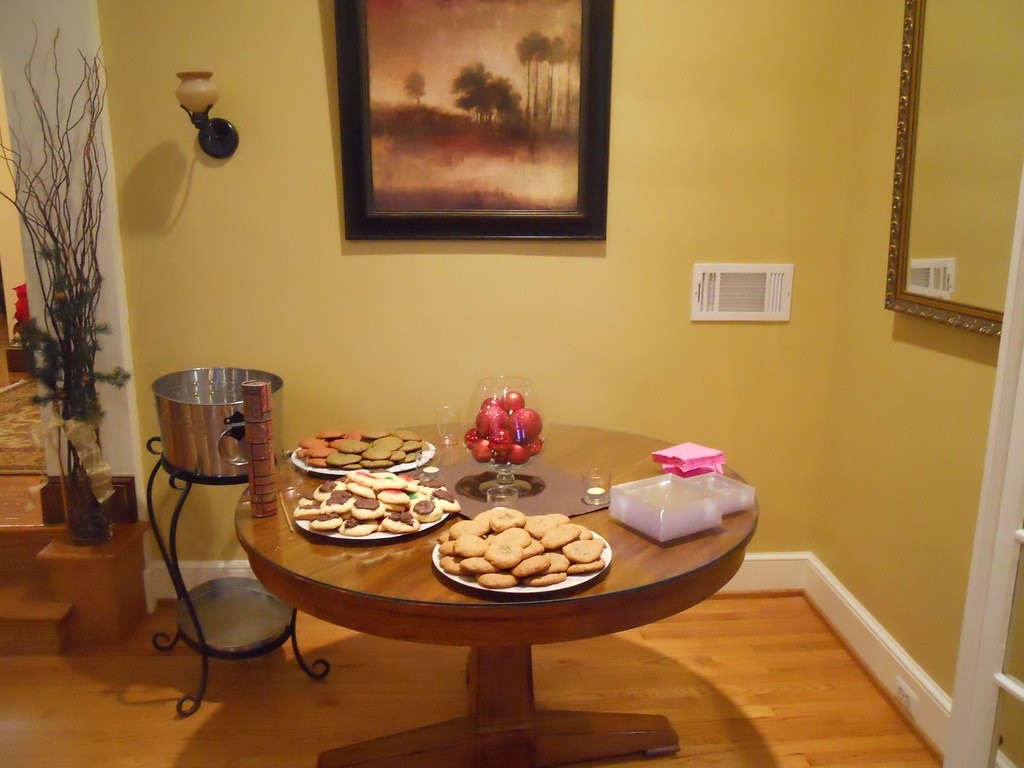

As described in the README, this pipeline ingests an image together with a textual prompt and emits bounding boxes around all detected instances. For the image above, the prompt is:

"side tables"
[146,436,332,716]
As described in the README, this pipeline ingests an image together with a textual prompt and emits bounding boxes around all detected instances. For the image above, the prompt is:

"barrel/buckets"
[150,366,285,475]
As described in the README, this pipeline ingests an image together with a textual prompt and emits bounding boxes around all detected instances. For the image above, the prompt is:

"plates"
[291,442,437,475]
[432,531,611,593]
[294,487,450,539]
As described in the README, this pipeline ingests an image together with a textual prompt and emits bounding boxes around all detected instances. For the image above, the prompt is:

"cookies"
[293,469,460,536]
[437,509,605,588]
[295,430,428,468]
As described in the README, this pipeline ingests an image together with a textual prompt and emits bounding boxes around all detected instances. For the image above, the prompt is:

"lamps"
[174,71,238,160]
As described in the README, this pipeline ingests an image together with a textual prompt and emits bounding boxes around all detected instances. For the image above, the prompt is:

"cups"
[582,468,610,504]
[415,449,442,483]
[436,404,462,446]
[487,488,517,509]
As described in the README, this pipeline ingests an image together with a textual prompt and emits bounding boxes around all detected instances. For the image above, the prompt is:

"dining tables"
[235,422,759,768]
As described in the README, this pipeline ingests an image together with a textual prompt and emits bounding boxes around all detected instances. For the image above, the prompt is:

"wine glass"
[462,376,547,498]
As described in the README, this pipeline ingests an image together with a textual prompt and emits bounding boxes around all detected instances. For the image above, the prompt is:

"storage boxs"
[608,473,722,542]
[685,471,756,516]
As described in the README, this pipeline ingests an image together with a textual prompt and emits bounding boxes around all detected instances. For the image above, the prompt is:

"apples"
[466,393,542,464]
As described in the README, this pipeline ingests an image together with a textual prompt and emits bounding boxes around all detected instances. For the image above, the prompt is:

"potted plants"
[0,13,132,546]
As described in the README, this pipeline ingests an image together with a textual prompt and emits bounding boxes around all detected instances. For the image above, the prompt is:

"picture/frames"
[885,0,1024,337]
[334,0,614,241]
